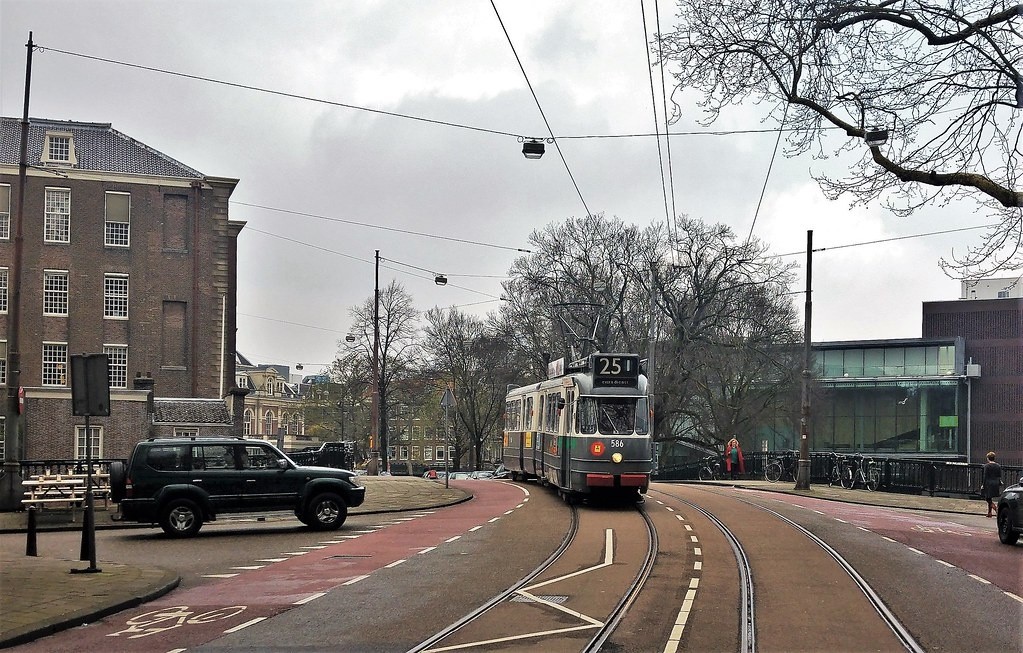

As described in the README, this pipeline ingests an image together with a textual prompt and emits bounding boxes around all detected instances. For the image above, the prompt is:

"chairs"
[223,450,234,465]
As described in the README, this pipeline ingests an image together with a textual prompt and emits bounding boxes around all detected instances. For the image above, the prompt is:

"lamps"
[345,334,356,343]
[521,138,546,160]
[295,364,304,370]
[434,274,447,286]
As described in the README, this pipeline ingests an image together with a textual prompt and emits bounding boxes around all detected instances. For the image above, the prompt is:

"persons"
[241,449,261,467]
[981,452,1001,517]
[726,438,745,480]
[426,468,437,479]
[223,447,235,466]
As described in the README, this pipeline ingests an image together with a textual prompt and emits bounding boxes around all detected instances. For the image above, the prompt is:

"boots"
[986,512,992,518]
[990,502,997,512]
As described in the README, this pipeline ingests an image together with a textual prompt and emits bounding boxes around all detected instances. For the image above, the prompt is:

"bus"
[501,301,654,508]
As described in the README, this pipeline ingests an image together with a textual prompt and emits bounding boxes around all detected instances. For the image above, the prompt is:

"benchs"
[20,485,112,502]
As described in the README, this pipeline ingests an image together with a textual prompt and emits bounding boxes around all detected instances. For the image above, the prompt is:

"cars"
[423,465,512,480]
[996,477,1023,545]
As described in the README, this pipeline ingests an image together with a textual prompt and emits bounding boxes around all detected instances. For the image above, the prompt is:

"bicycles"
[698,455,726,480]
[828,451,852,489]
[851,453,881,492]
[764,450,798,484]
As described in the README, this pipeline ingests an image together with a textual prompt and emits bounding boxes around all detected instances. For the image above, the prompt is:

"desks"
[21,479,84,522]
[30,474,110,511]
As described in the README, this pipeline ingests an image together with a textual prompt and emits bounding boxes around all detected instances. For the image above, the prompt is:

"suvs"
[109,436,366,538]
